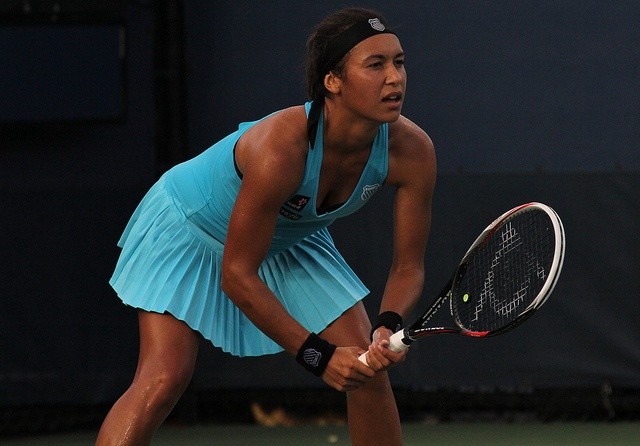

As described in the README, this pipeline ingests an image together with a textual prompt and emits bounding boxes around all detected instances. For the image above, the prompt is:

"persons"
[96,7,438,445]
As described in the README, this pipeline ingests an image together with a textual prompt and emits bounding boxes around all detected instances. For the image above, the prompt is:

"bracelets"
[293,330,337,378]
[367,310,410,342]
